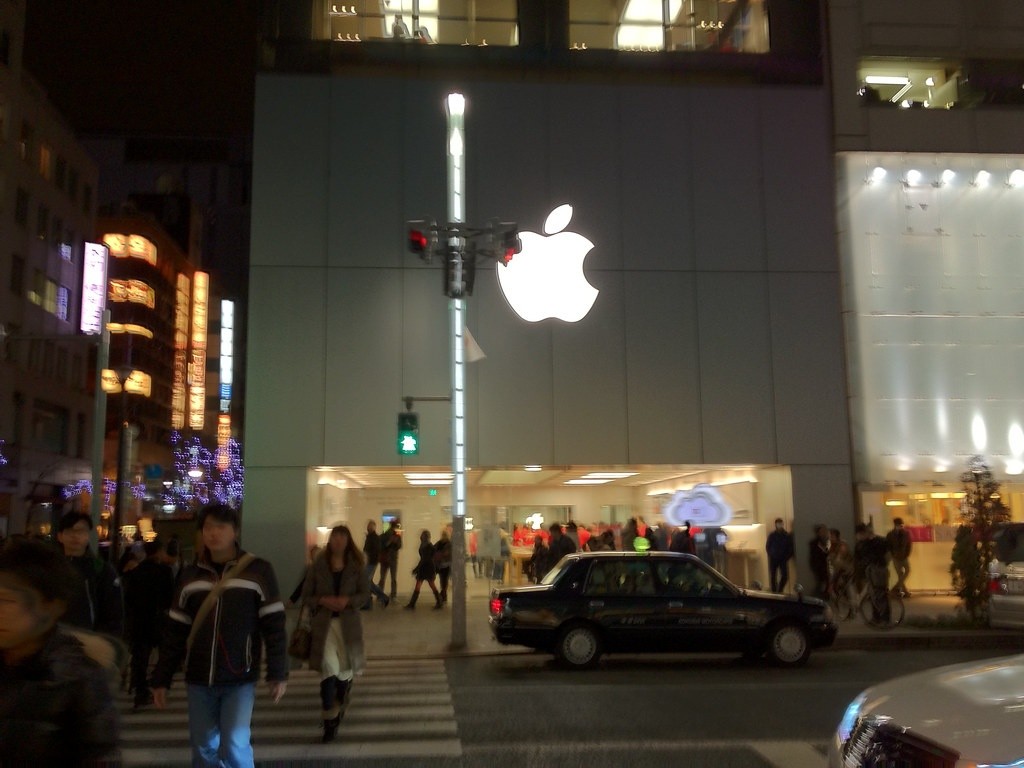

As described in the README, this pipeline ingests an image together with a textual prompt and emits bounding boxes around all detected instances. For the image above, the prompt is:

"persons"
[0,500,288,768]
[765,518,795,593]
[513,519,696,584]
[404,530,443,609]
[361,519,402,610]
[433,530,453,601]
[703,526,728,574]
[469,521,511,585]
[809,518,911,627]
[288,525,372,743]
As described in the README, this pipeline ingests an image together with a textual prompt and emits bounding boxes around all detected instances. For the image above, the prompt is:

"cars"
[825,652,1023,768]
[487,549,840,669]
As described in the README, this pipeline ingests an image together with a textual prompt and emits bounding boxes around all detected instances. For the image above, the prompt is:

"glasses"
[62,527,89,535]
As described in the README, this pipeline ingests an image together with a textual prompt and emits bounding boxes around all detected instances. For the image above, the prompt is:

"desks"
[505,549,534,585]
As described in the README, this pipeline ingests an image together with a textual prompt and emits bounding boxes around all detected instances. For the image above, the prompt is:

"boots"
[431,590,444,611]
[403,590,419,609]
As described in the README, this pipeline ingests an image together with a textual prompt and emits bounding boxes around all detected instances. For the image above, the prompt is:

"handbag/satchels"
[287,628,313,660]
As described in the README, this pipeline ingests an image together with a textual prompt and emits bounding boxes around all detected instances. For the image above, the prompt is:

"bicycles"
[817,560,905,630]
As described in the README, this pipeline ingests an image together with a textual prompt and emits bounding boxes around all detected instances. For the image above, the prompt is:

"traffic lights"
[398,411,420,455]
[492,218,522,269]
[406,218,435,262]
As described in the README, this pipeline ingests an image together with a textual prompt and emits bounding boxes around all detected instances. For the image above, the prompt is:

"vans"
[987,521,1024,630]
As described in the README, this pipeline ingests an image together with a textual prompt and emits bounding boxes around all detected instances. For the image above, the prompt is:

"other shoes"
[134,695,154,705]
[318,712,342,743]
[384,597,390,607]
[359,603,373,610]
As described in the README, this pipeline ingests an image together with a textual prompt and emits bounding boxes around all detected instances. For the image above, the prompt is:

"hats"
[549,523,563,530]
[894,518,904,524]
